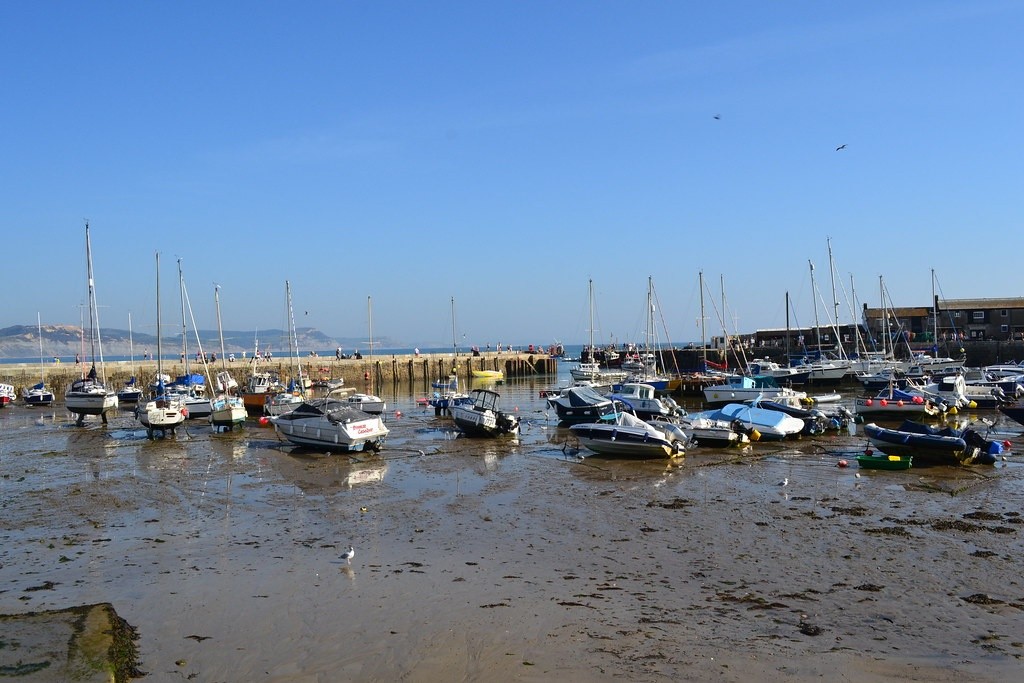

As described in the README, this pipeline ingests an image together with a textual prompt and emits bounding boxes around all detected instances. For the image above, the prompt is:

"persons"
[181,351,185,362]
[307,351,318,357]
[942,330,983,342]
[229,353,234,361]
[487,342,490,351]
[196,351,217,363]
[774,336,778,348]
[144,349,152,361]
[257,349,272,361]
[415,348,419,356]
[53,357,60,366]
[761,336,765,347]
[335,346,362,360]
[539,345,542,353]
[743,336,755,348]
[75,354,79,364]
[471,346,479,354]
[507,344,512,351]
[242,350,246,360]
[498,342,501,353]
[1009,331,1024,342]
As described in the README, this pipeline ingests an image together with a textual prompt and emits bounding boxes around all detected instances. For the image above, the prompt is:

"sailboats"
[265,280,313,416]
[743,236,968,392]
[238,333,288,409]
[63,222,119,414]
[208,284,245,432]
[22,311,56,406]
[571,268,760,394]
[430,296,475,408]
[118,310,145,401]
[137,253,189,429]
[171,255,214,419]
[346,295,389,414]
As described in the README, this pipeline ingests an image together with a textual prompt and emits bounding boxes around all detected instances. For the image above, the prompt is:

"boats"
[471,368,503,380]
[546,386,634,423]
[856,455,914,471]
[859,419,1002,466]
[700,377,809,404]
[602,347,620,366]
[432,380,458,389]
[568,361,601,380]
[812,394,842,403]
[921,361,1024,431]
[666,414,759,451]
[299,361,344,389]
[852,381,938,417]
[570,396,690,458]
[744,399,853,433]
[689,404,805,439]
[448,389,523,439]
[270,388,389,453]
[602,383,688,420]
[621,345,658,374]
[0,383,17,408]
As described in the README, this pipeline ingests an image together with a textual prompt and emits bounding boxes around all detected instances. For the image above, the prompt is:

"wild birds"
[462,334,467,339]
[304,310,308,315]
[835,143,849,152]
[772,477,789,488]
[337,546,355,564]
[713,115,720,120]
